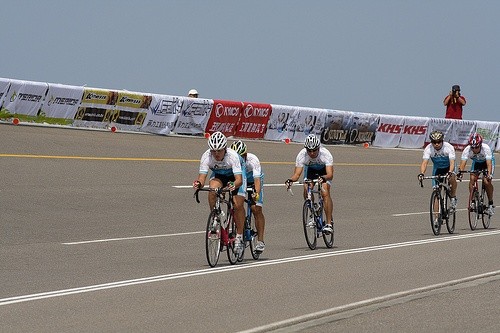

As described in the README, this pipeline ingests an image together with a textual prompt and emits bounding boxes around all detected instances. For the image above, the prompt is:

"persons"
[188,89,199,98]
[456,133,495,216]
[443,85,466,119]
[418,130,458,227]
[284,134,334,234]
[229,140,266,251]
[191,131,247,253]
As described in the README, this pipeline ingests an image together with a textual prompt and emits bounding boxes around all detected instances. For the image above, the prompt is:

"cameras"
[452,89,457,96]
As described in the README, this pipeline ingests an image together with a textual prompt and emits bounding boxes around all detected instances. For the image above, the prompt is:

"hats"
[188,89,199,97]
[452,86,460,91]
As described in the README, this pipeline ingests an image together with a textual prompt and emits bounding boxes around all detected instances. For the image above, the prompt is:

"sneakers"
[434,219,438,225]
[449,197,457,208]
[213,211,225,227]
[234,237,244,252]
[487,206,495,215]
[323,225,334,232]
[471,202,477,209]
[306,218,317,227]
[245,235,254,248]
[255,242,265,252]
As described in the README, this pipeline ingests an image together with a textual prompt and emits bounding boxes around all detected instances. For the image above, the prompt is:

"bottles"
[246,217,250,227]
[314,203,321,215]
[221,210,228,228]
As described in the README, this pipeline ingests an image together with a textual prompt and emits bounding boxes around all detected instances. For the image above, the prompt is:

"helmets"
[229,140,247,155]
[304,134,320,150]
[429,131,442,141]
[208,132,227,151]
[468,133,482,147]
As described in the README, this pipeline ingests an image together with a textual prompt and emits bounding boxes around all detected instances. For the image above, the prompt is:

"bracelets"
[456,96,460,99]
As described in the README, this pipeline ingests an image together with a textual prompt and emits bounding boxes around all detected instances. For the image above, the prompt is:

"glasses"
[471,146,480,149]
[211,149,225,153]
[432,140,443,145]
[308,148,319,153]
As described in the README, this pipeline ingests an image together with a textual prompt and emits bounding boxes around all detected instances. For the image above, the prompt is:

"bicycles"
[192,180,239,268]
[456,168,495,231]
[419,171,457,236]
[286,173,335,250]
[235,184,263,262]
